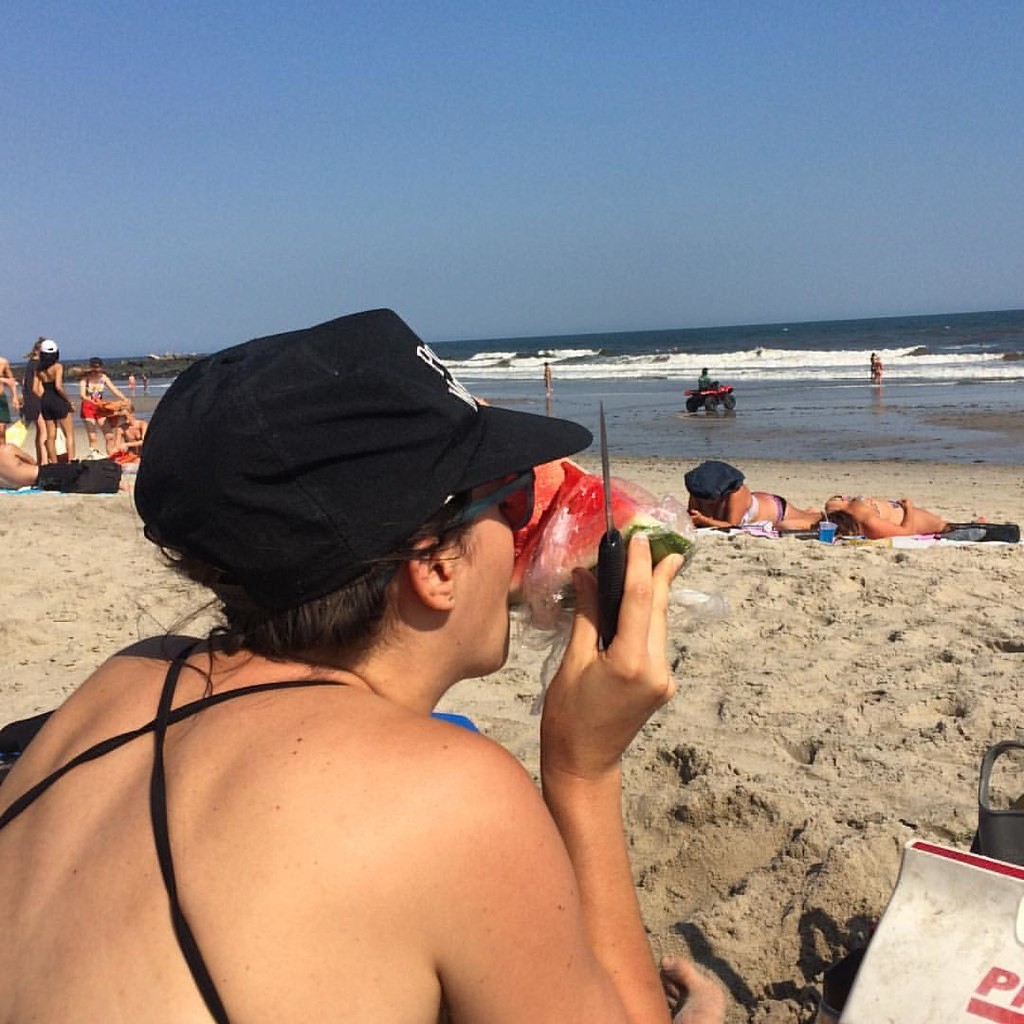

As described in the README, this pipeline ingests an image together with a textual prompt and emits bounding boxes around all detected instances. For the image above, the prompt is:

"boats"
[440,347,604,369]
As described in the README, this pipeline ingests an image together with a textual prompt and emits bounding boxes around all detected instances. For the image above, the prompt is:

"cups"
[820,522,839,544]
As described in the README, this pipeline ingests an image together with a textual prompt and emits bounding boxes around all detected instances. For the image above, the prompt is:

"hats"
[90,358,103,368]
[39,339,58,353]
[134,308,593,616]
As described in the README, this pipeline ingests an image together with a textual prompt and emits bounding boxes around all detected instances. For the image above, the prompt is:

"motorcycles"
[685,381,736,412]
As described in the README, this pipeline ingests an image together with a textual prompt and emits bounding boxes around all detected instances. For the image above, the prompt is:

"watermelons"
[509,459,695,610]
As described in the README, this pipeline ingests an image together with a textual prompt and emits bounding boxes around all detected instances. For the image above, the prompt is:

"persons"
[143,373,149,394]
[871,352,882,384]
[809,495,946,537]
[696,367,722,392]
[0,336,149,492]
[683,460,827,530]
[544,362,554,397]
[0,307,685,1024]
[129,372,135,395]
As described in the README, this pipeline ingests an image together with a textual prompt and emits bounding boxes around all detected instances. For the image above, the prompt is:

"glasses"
[441,467,536,534]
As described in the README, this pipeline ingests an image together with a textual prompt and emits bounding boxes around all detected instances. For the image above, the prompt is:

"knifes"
[598,399,625,653]
